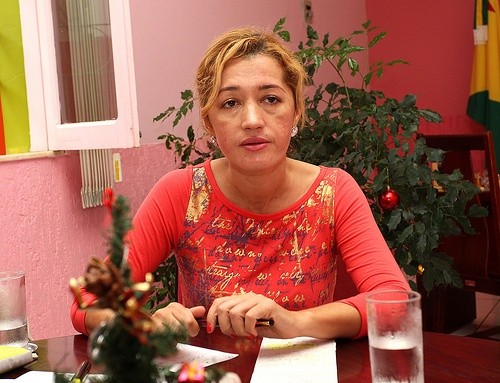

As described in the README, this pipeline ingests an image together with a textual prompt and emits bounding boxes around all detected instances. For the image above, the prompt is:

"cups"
[365,288,426,382]
[0,271,28,348]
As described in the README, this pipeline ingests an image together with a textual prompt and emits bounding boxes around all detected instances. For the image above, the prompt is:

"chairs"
[412,130,500,333]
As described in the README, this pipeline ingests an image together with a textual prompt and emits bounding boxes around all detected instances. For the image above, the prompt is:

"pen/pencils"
[200,319,272,330]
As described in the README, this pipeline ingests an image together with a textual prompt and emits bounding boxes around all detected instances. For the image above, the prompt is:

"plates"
[20,343,38,353]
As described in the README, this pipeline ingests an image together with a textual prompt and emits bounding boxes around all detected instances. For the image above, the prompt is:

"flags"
[466,0,500,176]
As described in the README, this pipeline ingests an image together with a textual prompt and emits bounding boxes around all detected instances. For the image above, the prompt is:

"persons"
[70,27,412,349]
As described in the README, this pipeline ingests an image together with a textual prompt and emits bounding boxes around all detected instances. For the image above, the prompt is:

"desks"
[1,326,499,383]
